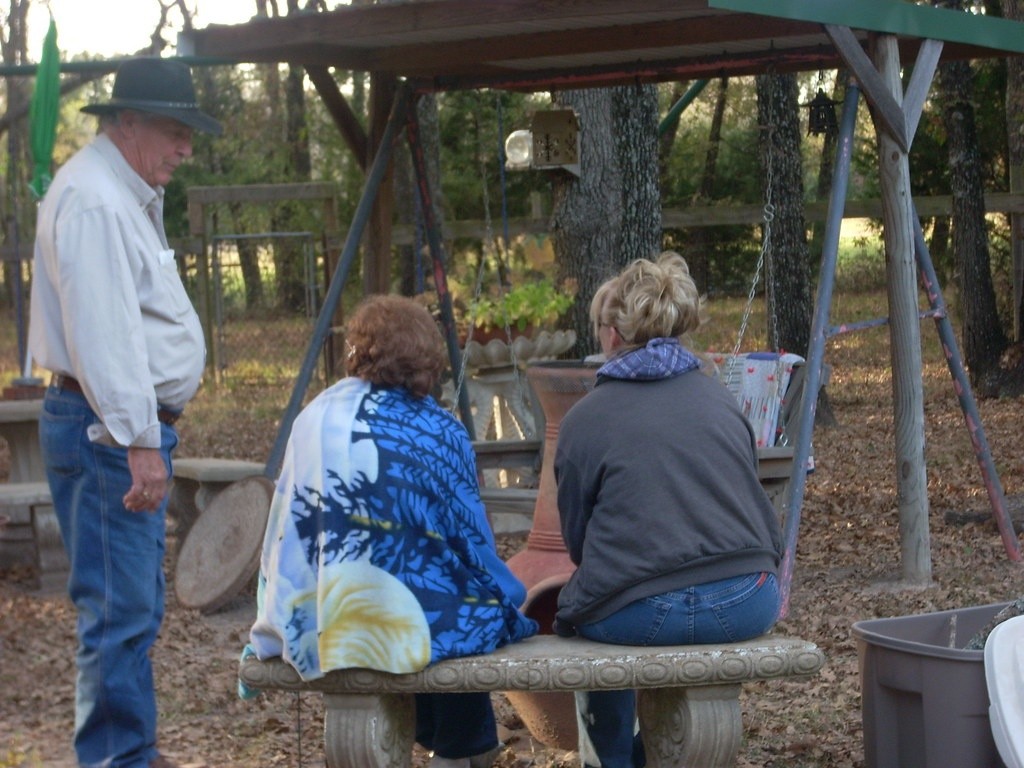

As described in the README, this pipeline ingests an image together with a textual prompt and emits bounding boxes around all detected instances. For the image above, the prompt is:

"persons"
[553,253,787,768]
[238,296,539,768]
[28,59,222,768]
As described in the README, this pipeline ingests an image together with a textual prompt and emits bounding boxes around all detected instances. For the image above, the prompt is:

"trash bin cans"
[848,600,1023,768]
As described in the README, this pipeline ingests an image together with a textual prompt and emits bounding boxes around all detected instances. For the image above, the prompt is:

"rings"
[140,491,150,502]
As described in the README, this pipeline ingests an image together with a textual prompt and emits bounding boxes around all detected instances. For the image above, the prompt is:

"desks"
[0,398,51,566]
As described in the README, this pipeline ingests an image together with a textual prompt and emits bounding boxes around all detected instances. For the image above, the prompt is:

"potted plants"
[455,279,577,351]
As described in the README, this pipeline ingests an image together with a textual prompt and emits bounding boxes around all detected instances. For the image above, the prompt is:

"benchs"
[0,482,61,588]
[239,636,825,768]
[168,458,266,537]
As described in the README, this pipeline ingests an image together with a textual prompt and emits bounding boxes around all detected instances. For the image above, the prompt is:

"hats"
[78,58,224,137]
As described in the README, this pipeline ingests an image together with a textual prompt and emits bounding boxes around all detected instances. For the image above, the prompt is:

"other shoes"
[428,752,471,768]
[469,742,505,768]
[149,756,210,768]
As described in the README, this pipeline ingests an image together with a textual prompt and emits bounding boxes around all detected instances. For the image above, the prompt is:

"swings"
[232,34,873,768]
[443,70,543,471]
[618,48,819,482]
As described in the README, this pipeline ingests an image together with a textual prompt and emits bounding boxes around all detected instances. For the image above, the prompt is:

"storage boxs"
[852,601,1024,768]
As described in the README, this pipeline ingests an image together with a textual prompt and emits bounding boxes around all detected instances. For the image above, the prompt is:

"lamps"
[800,69,843,139]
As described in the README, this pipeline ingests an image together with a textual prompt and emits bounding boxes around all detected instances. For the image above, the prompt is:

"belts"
[50,374,184,426]
